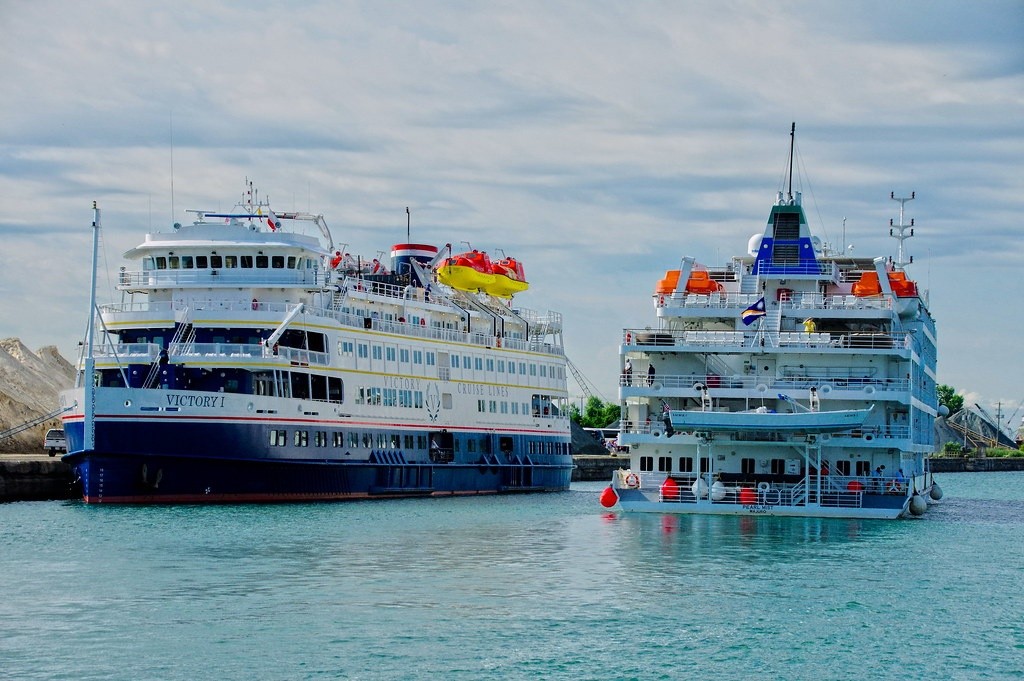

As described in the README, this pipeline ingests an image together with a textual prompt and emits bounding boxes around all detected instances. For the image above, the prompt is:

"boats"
[486,248,530,301]
[687,270,723,297]
[888,271,917,298]
[656,269,682,295]
[669,403,876,431]
[852,272,879,298]
[434,240,498,295]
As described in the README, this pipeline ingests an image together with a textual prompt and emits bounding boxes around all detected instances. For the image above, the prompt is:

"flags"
[662,400,672,411]
[331,254,345,270]
[258,209,264,223]
[373,263,387,276]
[741,297,767,325]
[267,210,281,230]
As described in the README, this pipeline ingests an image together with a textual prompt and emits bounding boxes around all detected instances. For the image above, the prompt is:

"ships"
[61,178,584,505]
[603,119,942,524]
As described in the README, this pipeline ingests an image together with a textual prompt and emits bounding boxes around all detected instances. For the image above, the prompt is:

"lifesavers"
[421,318,425,328]
[252,299,258,310]
[660,296,664,304]
[626,332,631,345]
[887,480,900,491]
[625,473,639,486]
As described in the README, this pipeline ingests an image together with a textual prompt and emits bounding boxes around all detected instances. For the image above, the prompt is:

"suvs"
[44,428,67,457]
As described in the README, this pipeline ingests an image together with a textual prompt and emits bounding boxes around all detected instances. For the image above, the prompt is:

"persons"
[623,358,634,386]
[872,467,883,493]
[646,364,658,387]
[803,316,817,337]
[536,406,552,417]
[892,468,904,493]
